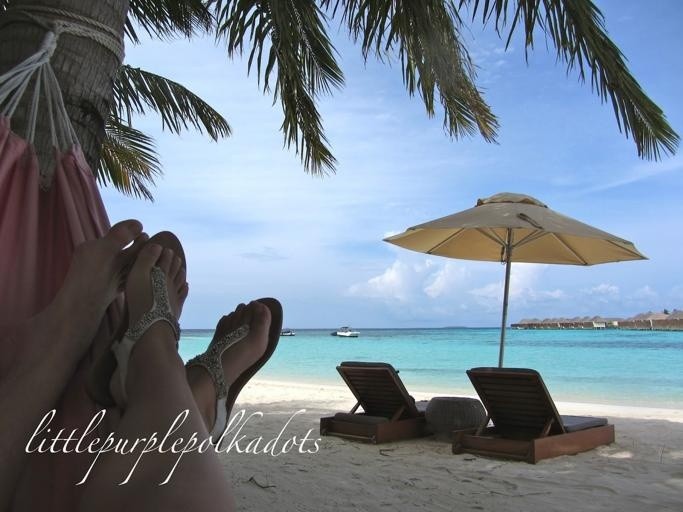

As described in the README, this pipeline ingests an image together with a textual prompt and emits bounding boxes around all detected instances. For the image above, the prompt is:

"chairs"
[319,361,437,445]
[453,367,614,463]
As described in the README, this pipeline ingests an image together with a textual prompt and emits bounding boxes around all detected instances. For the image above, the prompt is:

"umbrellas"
[382,191,649,370]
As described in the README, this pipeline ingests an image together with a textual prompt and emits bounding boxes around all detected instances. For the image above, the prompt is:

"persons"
[0,219,149,512]
[76,230,283,512]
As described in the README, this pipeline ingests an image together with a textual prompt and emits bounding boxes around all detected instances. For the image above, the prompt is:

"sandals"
[84,231,186,406]
[185,298,282,452]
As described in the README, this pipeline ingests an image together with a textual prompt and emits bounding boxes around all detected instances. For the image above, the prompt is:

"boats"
[279,329,295,336]
[330,326,359,337]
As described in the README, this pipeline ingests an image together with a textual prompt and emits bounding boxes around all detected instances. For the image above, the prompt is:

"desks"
[426,397,486,442]
